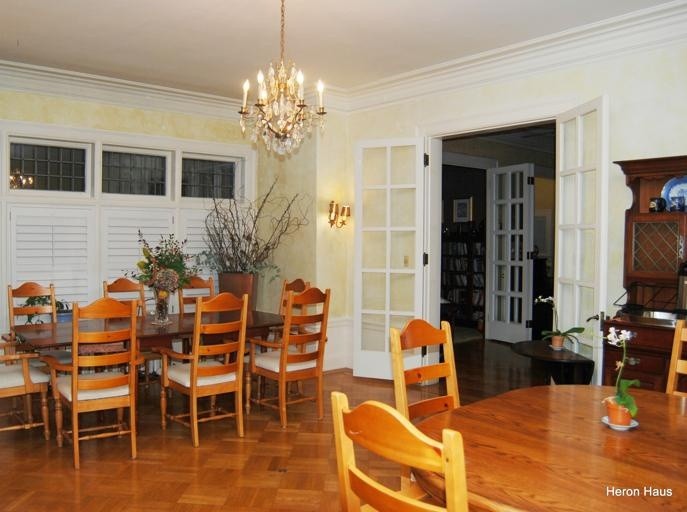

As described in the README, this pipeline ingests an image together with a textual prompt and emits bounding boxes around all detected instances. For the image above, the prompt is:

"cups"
[648,196,665,212]
[669,196,684,212]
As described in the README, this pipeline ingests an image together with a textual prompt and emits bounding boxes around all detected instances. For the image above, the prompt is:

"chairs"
[387,315,463,422]
[330,390,473,512]
[1,268,336,463]
[666,318,687,397]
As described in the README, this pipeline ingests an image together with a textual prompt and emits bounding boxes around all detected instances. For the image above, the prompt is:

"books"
[442,241,485,322]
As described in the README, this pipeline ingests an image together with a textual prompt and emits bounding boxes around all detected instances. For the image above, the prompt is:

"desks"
[513,339,596,388]
[408,383,687,512]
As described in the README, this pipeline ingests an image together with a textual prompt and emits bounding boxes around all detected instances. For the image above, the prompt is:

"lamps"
[326,198,351,228]
[235,0,329,157]
[9,166,35,188]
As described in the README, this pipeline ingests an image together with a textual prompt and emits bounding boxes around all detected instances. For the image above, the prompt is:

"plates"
[549,345,565,351]
[601,415,638,430]
[660,176,687,213]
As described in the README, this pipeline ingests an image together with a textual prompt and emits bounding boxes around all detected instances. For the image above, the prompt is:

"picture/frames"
[451,195,474,223]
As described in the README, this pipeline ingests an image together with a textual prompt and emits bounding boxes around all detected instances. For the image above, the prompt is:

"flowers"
[133,231,203,319]
[530,293,587,345]
[604,325,642,418]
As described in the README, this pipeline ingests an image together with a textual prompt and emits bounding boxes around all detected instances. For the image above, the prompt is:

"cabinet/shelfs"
[601,155,687,391]
[441,221,486,327]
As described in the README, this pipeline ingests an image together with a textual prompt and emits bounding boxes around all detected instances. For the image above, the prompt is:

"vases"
[600,393,632,426]
[552,333,564,347]
